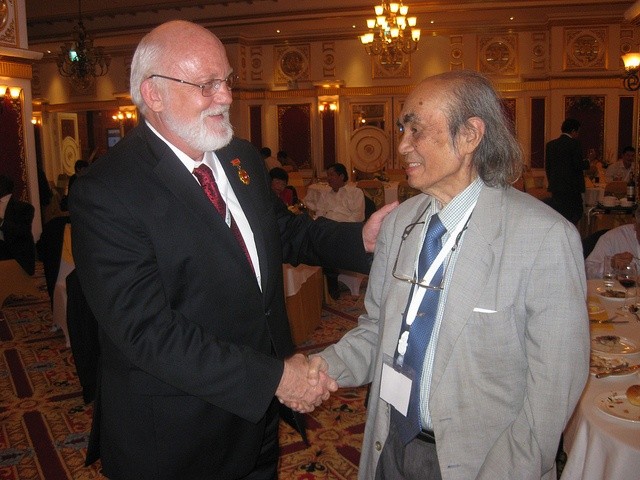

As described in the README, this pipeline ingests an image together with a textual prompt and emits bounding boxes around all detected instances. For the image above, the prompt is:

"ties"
[192,164,255,275]
[390,213,448,427]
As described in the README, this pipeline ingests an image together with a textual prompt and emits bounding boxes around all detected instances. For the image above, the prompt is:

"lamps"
[0,84,21,100]
[319,101,336,114]
[621,53,640,90]
[55,1,111,82]
[111,111,132,120]
[360,0,421,75]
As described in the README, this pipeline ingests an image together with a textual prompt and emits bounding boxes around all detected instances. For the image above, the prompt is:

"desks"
[304,182,400,220]
[581,209,640,240]
[583,173,610,205]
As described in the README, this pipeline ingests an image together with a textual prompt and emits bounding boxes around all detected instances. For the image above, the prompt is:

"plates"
[593,390,640,424]
[589,356,640,377]
[590,332,640,356]
[594,289,636,300]
[588,301,608,314]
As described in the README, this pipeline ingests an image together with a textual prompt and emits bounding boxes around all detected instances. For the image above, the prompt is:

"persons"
[261,147,280,173]
[606,146,636,182]
[585,208,639,279]
[69,160,88,193]
[583,146,606,188]
[277,150,287,165]
[545,118,590,227]
[313,163,365,223]
[269,168,294,206]
[36,196,71,294]
[0,175,13,241]
[278,73,591,478]
[68,21,399,478]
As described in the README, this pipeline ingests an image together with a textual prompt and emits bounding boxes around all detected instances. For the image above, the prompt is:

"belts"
[416,429,436,443]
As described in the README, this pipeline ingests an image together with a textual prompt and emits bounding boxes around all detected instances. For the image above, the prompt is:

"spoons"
[629,304,640,321]
[598,362,629,370]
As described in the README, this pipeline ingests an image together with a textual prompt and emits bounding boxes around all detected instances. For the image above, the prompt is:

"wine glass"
[298,201,306,215]
[617,262,638,311]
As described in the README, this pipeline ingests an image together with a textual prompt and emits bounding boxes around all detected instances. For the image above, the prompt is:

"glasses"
[392,221,468,290]
[148,72,239,98]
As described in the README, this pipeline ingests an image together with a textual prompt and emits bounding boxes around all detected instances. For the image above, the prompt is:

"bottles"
[626,171,635,201]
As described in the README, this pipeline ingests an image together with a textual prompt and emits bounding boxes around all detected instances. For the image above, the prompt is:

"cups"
[603,256,617,287]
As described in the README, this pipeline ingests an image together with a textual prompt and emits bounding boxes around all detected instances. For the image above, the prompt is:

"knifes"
[589,318,629,324]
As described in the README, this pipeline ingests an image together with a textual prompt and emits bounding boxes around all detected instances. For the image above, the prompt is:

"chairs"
[1,199,35,304]
[338,196,377,298]
[37,218,76,348]
